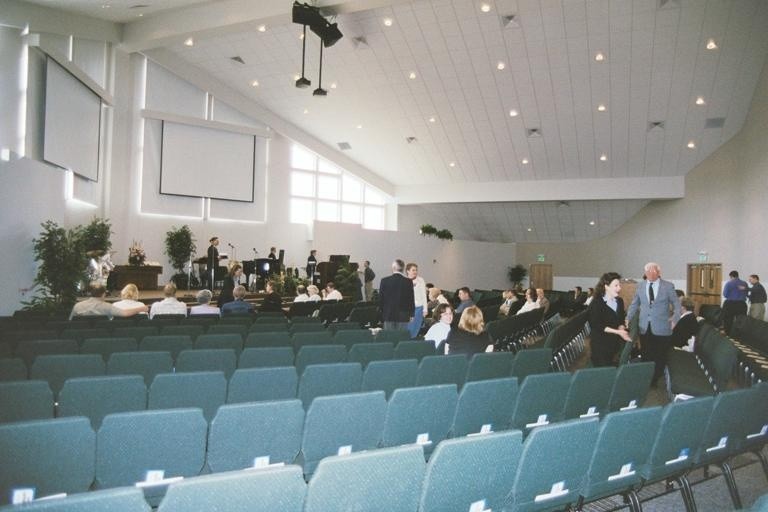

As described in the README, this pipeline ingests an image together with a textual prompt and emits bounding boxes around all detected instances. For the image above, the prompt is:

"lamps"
[296,24,328,97]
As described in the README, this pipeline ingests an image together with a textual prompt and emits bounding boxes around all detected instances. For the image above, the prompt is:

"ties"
[649,282,654,304]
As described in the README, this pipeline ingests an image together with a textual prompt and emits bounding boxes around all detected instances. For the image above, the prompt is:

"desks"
[108,266,163,289]
[315,262,359,289]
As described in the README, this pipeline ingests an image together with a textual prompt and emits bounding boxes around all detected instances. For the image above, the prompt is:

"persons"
[376,259,428,338]
[719,271,749,334]
[189,288,221,315]
[587,272,632,369]
[738,275,767,322]
[624,262,681,389]
[423,283,494,355]
[671,297,698,348]
[306,249,317,279]
[221,285,256,314]
[69,284,150,322]
[268,247,276,258]
[217,264,243,307]
[295,282,343,302]
[206,236,219,291]
[363,260,375,301]
[257,281,282,311]
[565,286,595,319]
[149,283,188,320]
[112,283,149,319]
[499,287,550,317]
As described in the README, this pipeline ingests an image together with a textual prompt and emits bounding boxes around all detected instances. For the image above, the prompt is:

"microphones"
[228,243,234,248]
[253,248,258,253]
